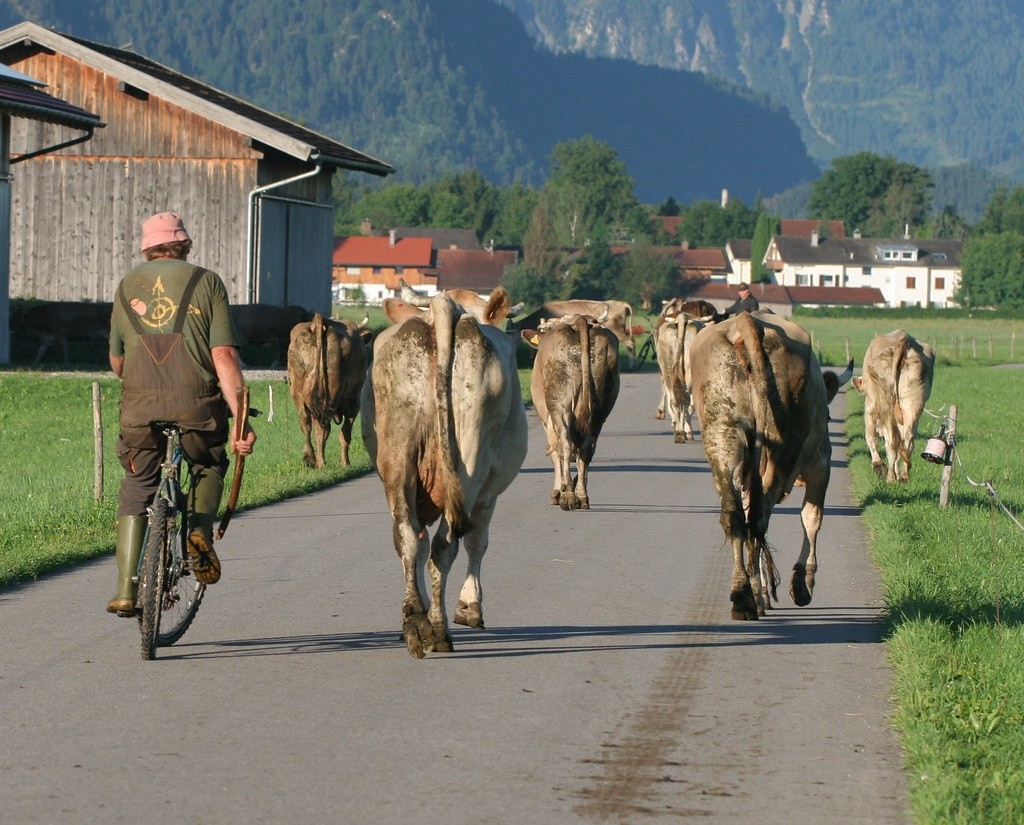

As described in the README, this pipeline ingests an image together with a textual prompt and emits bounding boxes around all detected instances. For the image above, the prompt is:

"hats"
[141,212,191,252]
[736,283,748,291]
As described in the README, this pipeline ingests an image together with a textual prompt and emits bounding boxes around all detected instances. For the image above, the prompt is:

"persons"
[102,211,257,613]
[724,281,759,317]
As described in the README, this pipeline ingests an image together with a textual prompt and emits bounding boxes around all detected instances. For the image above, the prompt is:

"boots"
[186,469,224,584]
[105,515,148,614]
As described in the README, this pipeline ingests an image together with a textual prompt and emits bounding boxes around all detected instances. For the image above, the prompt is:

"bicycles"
[116,407,265,660]
[633,314,658,373]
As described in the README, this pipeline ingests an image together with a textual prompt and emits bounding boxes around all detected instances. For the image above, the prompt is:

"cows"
[852,327,935,483]
[362,278,527,657]
[520,305,620,512]
[688,308,854,620]
[505,298,634,372]
[288,310,369,469]
[654,297,718,443]
